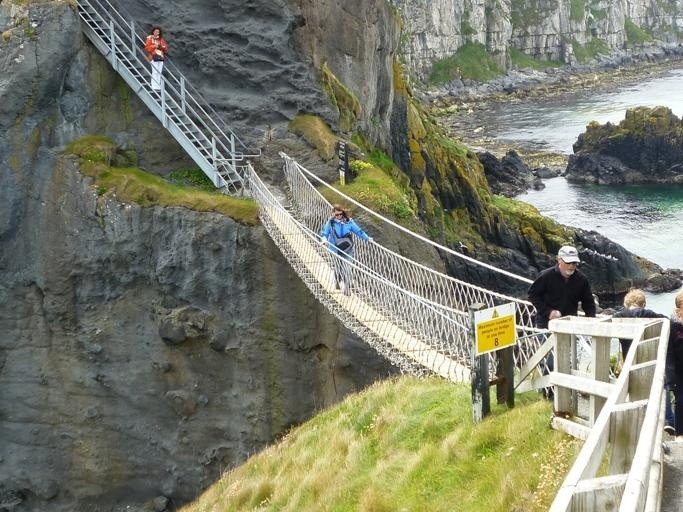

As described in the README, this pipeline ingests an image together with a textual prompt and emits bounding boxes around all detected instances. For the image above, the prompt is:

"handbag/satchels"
[154,55,164,61]
[335,238,353,252]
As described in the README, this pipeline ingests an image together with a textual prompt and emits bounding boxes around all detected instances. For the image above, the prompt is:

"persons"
[668,290,682,438]
[610,289,674,436]
[320,205,372,296]
[144,26,169,90]
[527,246,596,400]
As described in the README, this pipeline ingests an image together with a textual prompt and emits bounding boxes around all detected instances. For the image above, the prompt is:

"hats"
[558,246,580,263]
